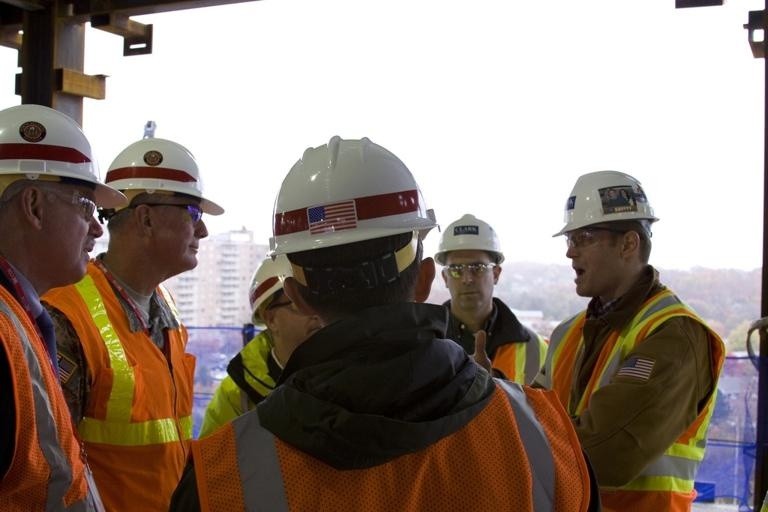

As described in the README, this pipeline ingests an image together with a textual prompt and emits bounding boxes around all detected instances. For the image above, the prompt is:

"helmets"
[248,255,294,325]
[552,171,660,237]
[104,137,225,216]
[269,136,436,253]
[433,214,506,266]
[0,104,129,209]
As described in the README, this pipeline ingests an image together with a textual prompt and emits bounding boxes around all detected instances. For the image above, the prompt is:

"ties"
[38,307,58,380]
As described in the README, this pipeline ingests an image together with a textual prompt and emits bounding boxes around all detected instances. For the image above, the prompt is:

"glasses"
[567,228,625,247]
[3,184,97,221]
[267,300,301,314]
[130,202,204,221]
[443,262,498,278]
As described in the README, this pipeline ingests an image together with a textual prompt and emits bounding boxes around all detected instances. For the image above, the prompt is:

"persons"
[466,170,724,512]
[434,212,549,386]
[198,255,323,437]
[39,136,224,511]
[169,137,592,512]
[0,103,127,512]
